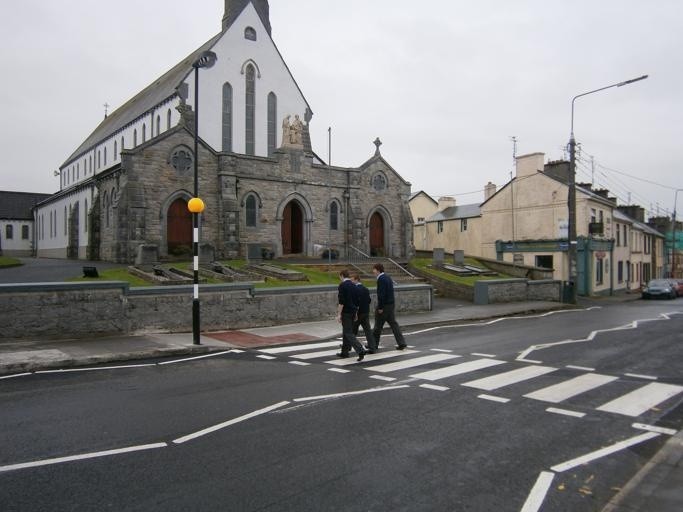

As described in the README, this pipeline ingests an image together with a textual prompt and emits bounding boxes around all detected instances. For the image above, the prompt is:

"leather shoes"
[336,343,379,361]
[395,343,408,350]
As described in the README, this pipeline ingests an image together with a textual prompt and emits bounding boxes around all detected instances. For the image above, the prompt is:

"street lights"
[191,50,218,345]
[568,75,649,281]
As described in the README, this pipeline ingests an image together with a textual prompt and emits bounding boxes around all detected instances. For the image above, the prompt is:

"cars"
[642,279,678,300]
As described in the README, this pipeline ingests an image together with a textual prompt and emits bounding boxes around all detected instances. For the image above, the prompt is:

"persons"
[335,270,378,360]
[366,264,408,350]
